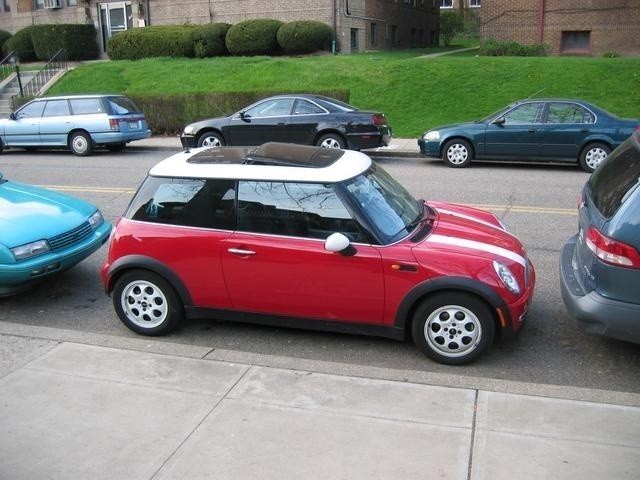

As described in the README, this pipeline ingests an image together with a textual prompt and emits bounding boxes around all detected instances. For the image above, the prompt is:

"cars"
[1,93,153,157]
[179,92,394,154]
[555,123,640,350]
[414,95,640,173]
[99,138,538,369]
[0,171,115,300]
